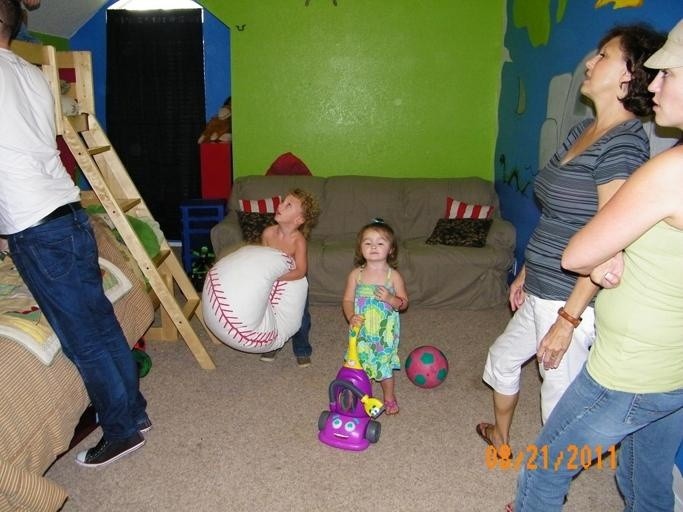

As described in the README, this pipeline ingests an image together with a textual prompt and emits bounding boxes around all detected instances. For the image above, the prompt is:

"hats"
[642,17,683,71]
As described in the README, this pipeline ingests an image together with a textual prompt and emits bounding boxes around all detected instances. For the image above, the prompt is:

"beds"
[0,36,225,511]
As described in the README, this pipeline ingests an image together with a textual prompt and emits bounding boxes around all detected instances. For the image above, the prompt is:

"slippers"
[475,421,513,460]
[383,394,399,415]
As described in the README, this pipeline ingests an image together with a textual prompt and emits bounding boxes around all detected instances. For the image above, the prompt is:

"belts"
[24,201,82,231]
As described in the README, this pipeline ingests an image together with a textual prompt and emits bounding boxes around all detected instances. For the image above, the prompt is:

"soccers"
[405,347,447,389]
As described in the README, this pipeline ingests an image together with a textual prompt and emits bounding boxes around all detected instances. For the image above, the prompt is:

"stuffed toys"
[197,96,231,142]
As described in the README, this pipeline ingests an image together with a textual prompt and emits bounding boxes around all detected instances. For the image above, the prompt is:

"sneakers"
[74,430,147,467]
[296,354,310,367]
[133,413,152,433]
[259,350,277,362]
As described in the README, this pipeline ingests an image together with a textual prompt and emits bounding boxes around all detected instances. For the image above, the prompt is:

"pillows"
[425,215,494,248]
[237,194,282,216]
[444,195,496,224]
[234,208,279,243]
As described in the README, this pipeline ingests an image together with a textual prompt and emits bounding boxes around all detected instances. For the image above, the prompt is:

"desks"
[178,195,227,280]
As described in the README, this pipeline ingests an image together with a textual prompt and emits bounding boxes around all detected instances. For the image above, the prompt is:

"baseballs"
[201,246,308,353]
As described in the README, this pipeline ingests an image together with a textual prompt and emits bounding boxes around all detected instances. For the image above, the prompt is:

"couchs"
[204,173,521,314]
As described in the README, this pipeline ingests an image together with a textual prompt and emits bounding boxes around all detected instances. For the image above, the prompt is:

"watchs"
[558,307,582,328]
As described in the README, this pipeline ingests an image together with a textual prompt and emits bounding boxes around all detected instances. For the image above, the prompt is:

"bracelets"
[392,296,403,313]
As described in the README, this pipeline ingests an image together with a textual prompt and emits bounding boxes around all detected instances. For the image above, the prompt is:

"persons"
[476,20,669,462]
[509,18,683,512]
[259,188,323,368]
[0,0,152,468]
[343,214,409,415]
